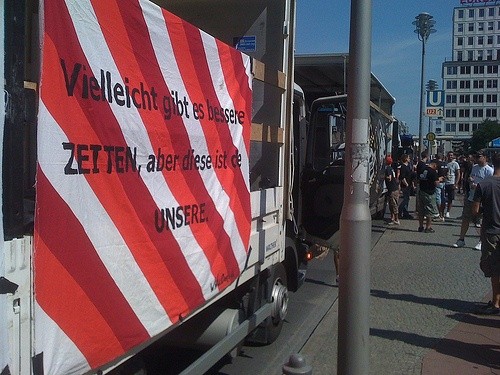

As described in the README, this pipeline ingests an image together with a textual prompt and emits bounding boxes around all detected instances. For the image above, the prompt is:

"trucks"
[4,0,402,375]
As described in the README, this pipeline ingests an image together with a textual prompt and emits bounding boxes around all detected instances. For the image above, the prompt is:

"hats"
[386,157,392,165]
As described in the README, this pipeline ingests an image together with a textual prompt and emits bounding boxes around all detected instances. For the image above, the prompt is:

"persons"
[384,156,400,225]
[334,250,340,283]
[398,153,414,219]
[415,159,443,232]
[473,151,500,313]
[453,149,494,250]
[416,152,473,222]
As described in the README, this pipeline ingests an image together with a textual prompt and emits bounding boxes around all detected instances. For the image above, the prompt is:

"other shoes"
[480,305,500,316]
[452,239,465,248]
[403,213,414,219]
[398,214,404,219]
[432,216,445,222]
[418,226,424,232]
[475,223,481,228]
[445,212,450,218]
[472,240,482,251]
[384,219,400,225]
[425,227,436,233]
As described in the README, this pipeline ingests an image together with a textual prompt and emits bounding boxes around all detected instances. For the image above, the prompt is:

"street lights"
[412,11,437,162]
[423,77,439,160]
